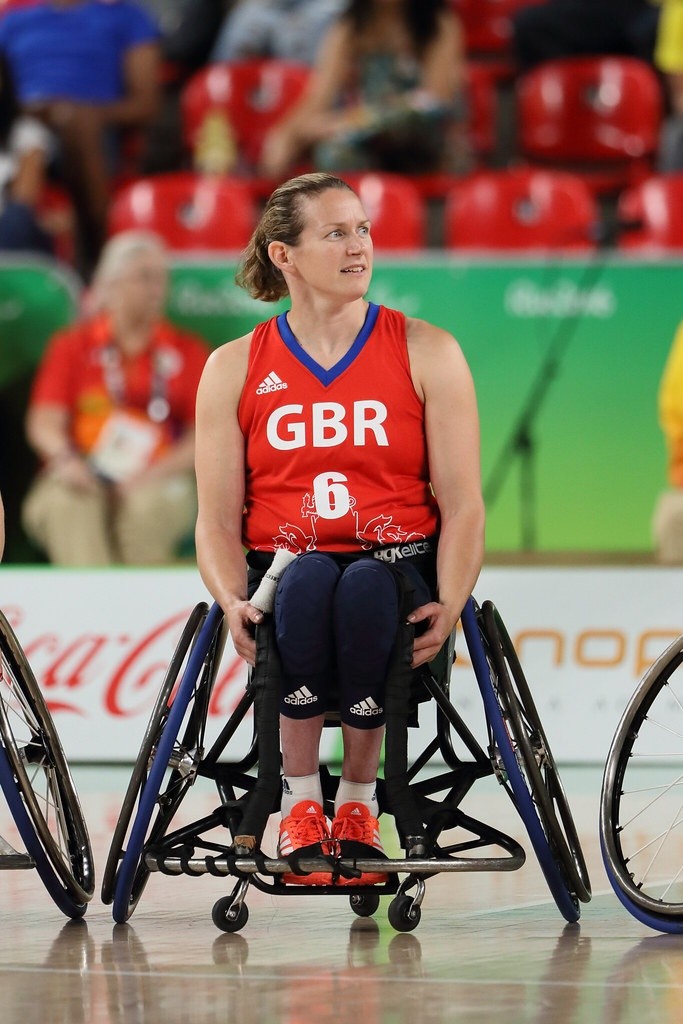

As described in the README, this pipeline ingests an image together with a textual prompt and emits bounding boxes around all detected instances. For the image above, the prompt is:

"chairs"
[513,54,662,194]
[443,166,598,252]
[613,172,682,251]
[180,59,314,204]
[104,170,256,251]
[318,170,427,251]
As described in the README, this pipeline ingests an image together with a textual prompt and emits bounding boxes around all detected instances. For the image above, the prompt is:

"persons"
[22,235,210,566]
[0,0,348,262]
[193,178,486,885]
[262,0,473,180]
[481,0,672,245]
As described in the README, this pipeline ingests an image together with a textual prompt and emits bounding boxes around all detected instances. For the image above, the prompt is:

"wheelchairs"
[100,596,594,928]
[597,631,682,933]
[0,608,95,922]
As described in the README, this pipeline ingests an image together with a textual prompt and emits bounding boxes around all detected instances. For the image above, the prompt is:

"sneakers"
[278,799,331,889]
[332,801,387,888]
